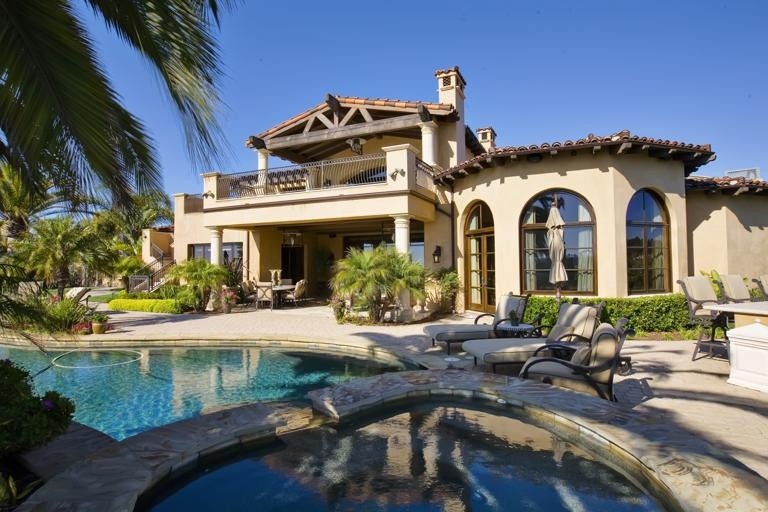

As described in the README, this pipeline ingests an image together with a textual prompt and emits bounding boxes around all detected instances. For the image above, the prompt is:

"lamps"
[432,246,442,263]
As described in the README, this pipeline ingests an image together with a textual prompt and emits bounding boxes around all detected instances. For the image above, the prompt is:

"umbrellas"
[545,202,569,309]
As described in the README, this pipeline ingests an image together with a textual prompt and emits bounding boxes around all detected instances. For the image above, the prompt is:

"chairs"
[63,285,98,310]
[242,278,309,311]
[676,272,767,362]
[422,291,638,407]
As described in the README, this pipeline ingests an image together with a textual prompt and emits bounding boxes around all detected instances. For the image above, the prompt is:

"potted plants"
[89,314,112,334]
